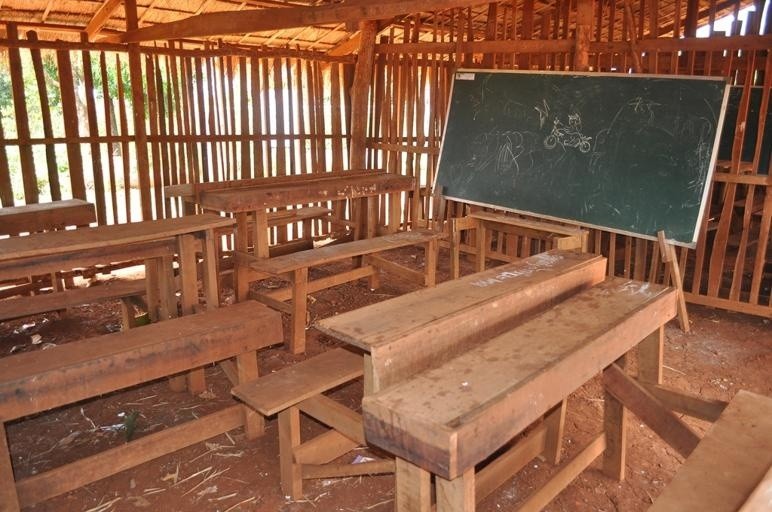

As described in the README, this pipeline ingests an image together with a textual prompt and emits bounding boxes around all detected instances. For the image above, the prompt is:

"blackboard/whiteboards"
[430,68,732,249]
[715,87,772,175]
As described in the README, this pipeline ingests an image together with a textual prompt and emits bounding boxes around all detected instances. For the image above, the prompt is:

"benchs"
[0,168,772,512]
